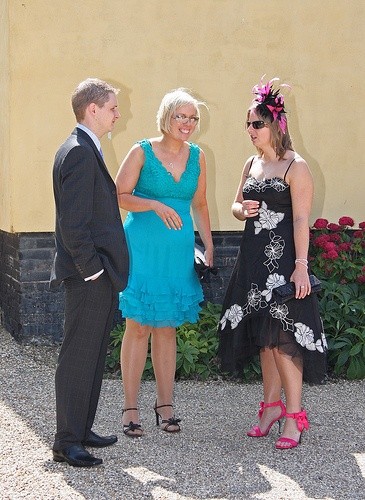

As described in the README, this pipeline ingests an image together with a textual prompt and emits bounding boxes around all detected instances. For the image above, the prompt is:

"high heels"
[275,407,310,449]
[121,408,143,437]
[153,398,181,433]
[246,400,287,437]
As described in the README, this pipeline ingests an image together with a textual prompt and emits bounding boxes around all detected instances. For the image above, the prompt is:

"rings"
[301,286,304,288]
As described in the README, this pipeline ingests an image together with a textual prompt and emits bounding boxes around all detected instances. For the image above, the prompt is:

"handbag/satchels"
[194,242,210,272]
[272,275,323,306]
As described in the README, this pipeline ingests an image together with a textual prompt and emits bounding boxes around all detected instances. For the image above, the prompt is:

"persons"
[216,77,328,449]
[115,90,213,437]
[51,79,129,467]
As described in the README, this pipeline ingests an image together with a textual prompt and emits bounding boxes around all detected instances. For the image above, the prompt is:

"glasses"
[247,121,264,129]
[172,113,200,123]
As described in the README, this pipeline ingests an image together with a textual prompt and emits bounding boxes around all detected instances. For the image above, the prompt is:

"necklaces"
[169,162,174,169]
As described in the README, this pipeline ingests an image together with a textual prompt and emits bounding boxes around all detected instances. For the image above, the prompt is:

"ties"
[99,147,103,157]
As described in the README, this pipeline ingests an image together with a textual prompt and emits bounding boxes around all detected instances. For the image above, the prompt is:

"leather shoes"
[52,445,103,467]
[84,431,118,447]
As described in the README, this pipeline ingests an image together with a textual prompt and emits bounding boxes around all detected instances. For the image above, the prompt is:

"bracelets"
[295,259,309,269]
